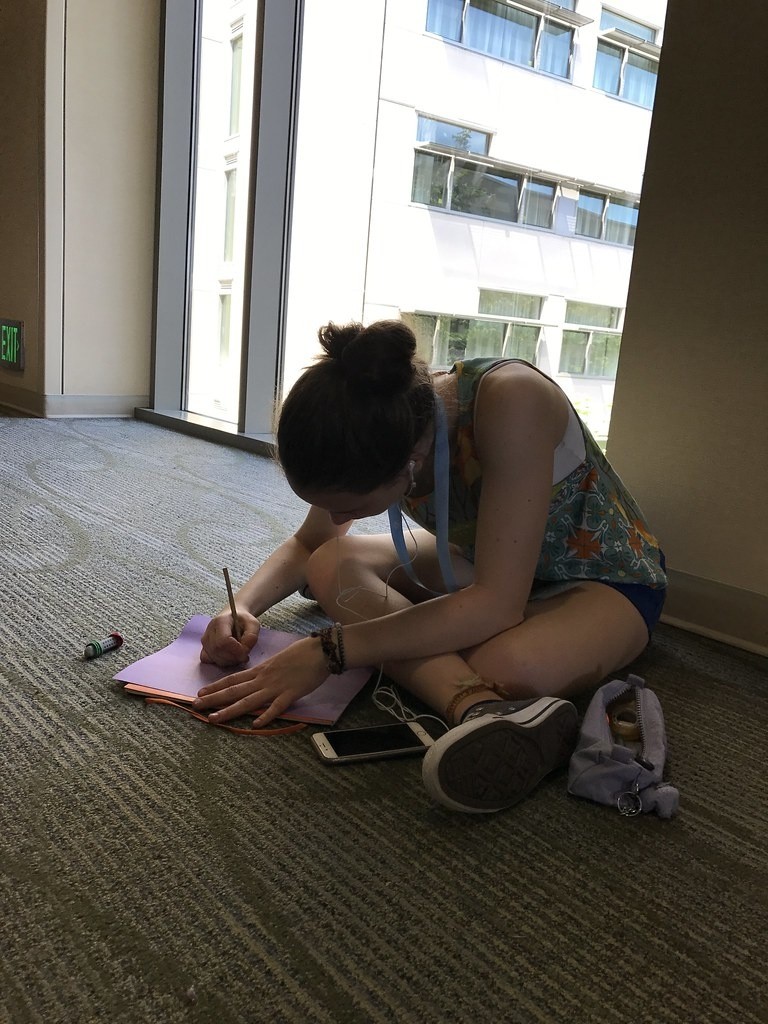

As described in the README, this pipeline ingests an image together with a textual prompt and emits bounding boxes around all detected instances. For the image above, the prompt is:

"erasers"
[84,632,123,661]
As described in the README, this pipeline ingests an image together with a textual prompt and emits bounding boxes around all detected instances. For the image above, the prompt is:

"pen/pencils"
[222,567,242,642]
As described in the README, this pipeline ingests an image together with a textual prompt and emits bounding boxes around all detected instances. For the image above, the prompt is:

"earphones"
[408,459,415,479]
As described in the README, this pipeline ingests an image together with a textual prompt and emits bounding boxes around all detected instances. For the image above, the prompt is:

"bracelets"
[310,623,347,676]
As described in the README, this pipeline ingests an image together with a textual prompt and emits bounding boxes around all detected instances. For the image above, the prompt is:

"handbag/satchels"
[553,670,679,820]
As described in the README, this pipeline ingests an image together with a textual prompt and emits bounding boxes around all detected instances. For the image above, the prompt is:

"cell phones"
[311,721,435,766]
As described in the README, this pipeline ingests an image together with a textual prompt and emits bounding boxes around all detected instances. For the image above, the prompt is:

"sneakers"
[422,695,572,814]
[298,583,315,601]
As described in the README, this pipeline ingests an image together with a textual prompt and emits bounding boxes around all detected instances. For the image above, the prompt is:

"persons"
[191,321,670,815]
